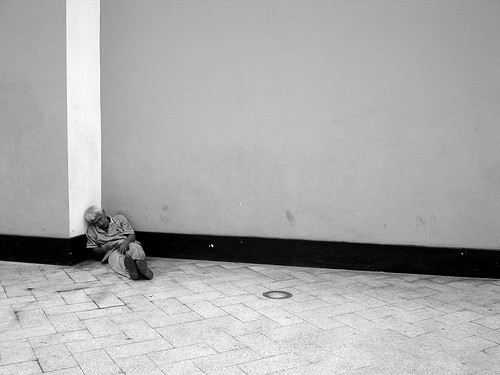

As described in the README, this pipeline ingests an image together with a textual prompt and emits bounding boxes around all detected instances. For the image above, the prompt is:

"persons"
[84,206,153,281]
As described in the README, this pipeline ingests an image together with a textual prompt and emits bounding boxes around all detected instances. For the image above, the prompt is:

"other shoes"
[124,257,138,280]
[136,259,153,279]
